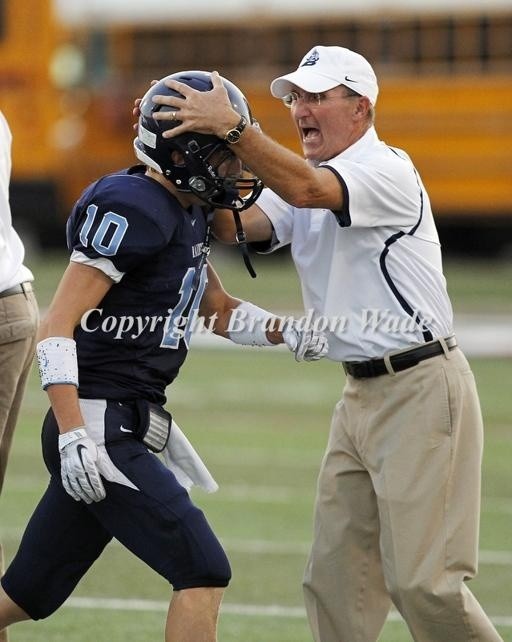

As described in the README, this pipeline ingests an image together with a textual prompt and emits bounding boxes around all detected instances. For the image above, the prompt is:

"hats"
[270,44,378,106]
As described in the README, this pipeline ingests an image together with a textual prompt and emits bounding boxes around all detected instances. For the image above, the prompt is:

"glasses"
[281,91,359,108]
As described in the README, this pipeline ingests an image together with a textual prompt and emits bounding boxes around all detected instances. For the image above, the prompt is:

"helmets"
[133,69,251,205]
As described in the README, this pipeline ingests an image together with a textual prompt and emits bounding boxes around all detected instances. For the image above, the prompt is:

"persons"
[0,110,40,495]
[132,45,505,642]
[0,71,252,642]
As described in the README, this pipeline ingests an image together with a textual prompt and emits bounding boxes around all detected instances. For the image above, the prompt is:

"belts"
[341,335,458,380]
[0,281,33,299]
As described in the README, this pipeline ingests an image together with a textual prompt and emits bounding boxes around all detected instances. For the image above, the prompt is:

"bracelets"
[36,337,79,391]
[229,302,278,346]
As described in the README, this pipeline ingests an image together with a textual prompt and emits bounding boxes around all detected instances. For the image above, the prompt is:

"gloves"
[58,426,115,505]
[281,318,329,364]
